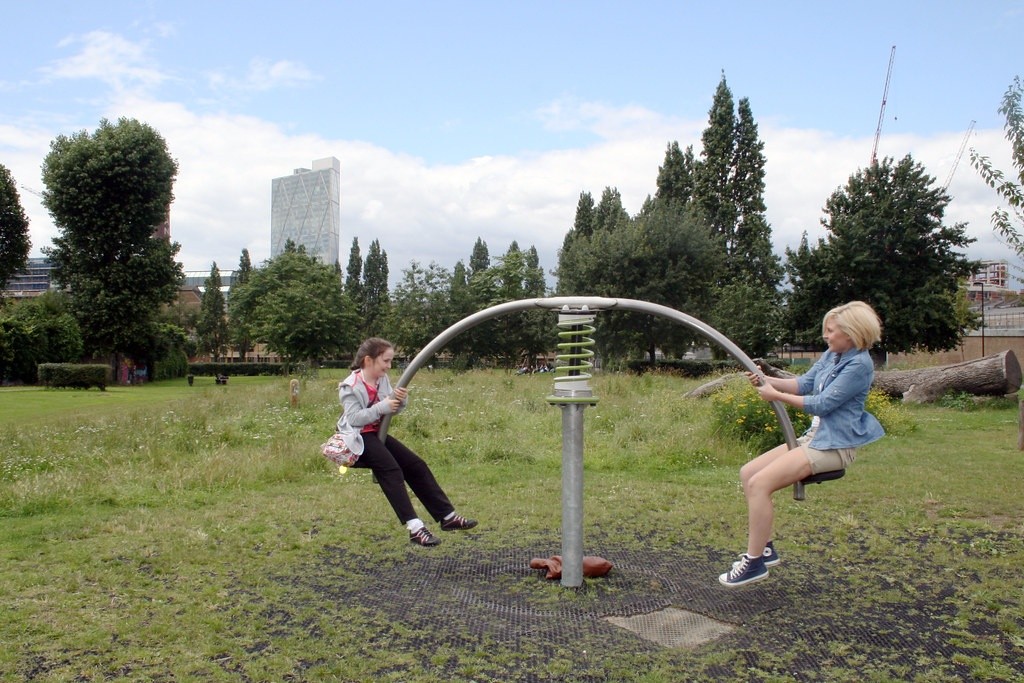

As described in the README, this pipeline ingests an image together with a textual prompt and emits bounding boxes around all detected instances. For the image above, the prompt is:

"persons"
[718,300,887,587]
[338,338,478,548]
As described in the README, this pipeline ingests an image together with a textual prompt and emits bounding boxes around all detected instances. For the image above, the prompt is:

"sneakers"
[409,526,442,548]
[440,513,479,531]
[718,539,782,588]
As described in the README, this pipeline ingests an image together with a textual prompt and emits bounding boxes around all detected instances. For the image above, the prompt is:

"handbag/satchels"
[323,434,359,467]
[529,555,615,580]
